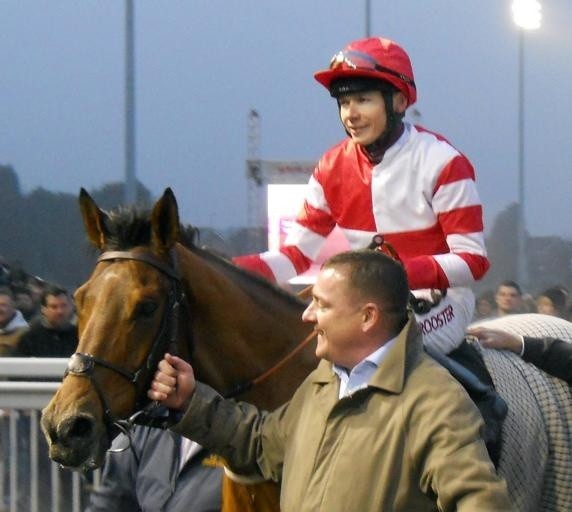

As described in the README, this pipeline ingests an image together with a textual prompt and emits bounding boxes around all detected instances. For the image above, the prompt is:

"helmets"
[314,37,417,108]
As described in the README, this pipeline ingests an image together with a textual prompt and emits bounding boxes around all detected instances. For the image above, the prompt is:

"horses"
[40,185,572,512]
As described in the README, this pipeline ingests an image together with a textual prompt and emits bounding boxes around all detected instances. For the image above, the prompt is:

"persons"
[238,38,490,352]
[465,280,572,325]
[148,247,520,512]
[1,259,80,357]
[464,327,572,384]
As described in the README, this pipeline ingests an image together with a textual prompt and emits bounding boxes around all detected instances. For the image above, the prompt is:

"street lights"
[509,0,543,291]
